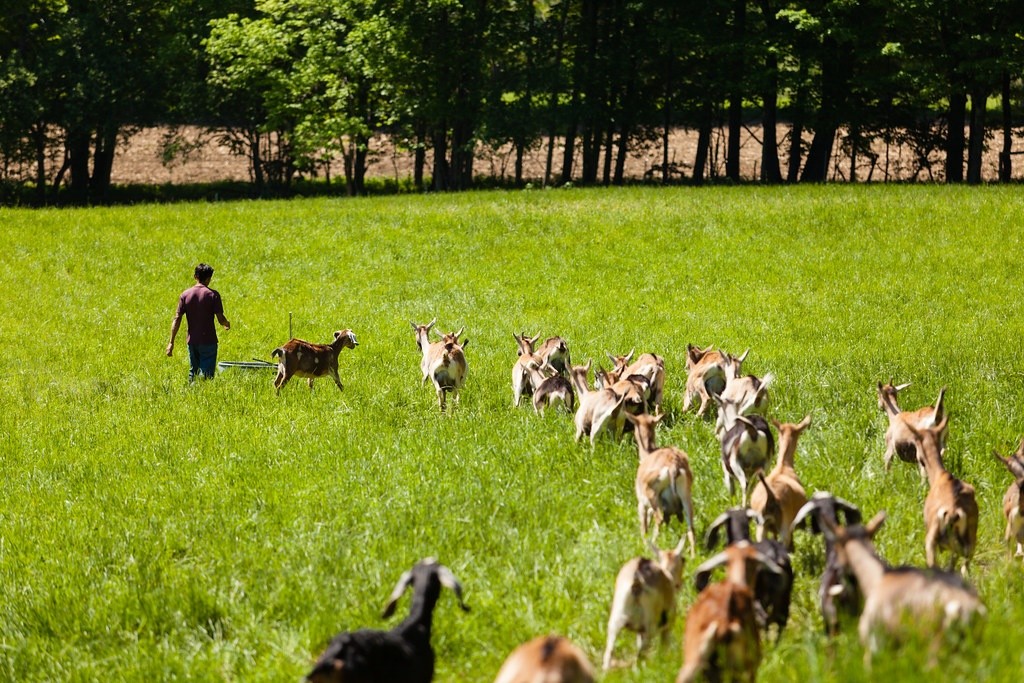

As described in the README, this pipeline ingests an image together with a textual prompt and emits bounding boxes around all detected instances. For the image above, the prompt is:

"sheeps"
[297,558,476,683]
[992,439,1024,557]
[512,330,663,449]
[271,329,359,396]
[495,342,985,683]
[408,317,469,413]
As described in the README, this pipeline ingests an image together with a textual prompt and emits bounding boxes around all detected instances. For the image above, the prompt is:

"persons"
[166,263,231,384]
